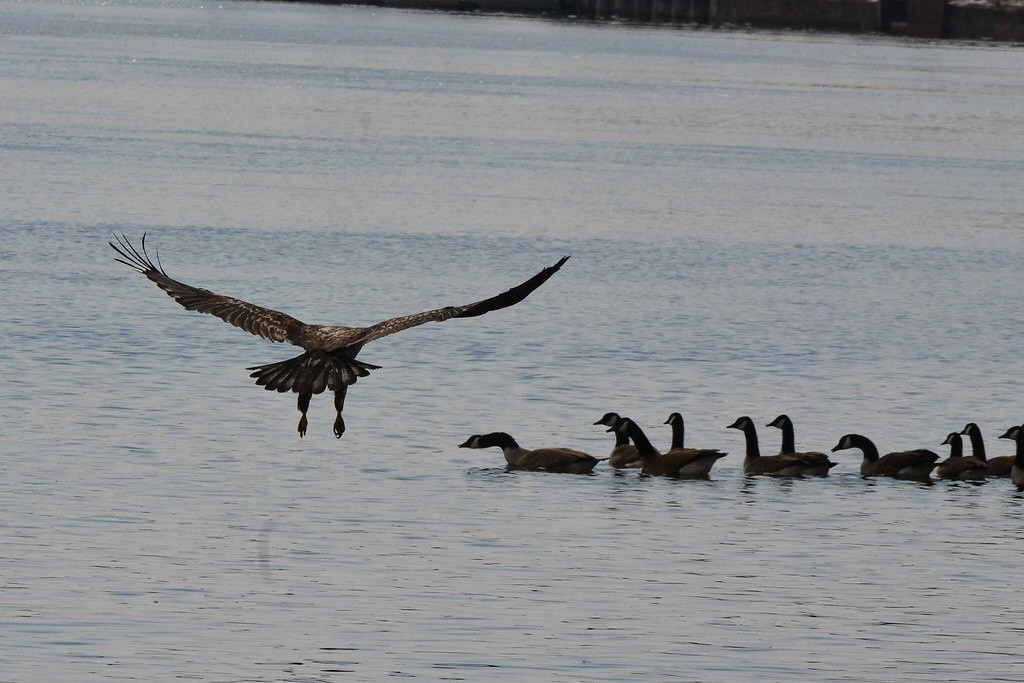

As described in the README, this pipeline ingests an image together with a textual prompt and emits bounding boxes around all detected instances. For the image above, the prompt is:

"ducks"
[457,412,1024,490]
